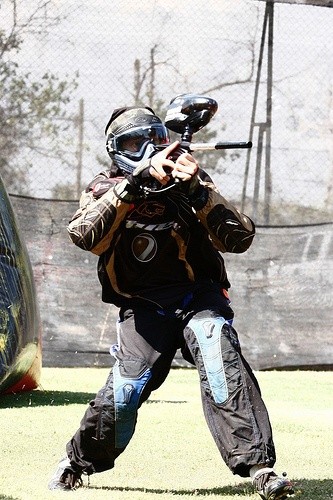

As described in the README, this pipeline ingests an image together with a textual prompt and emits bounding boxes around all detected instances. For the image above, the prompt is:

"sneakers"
[250,467,294,500]
[49,455,84,493]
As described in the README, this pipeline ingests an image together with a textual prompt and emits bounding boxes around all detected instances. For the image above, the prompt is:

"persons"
[48,103,295,500]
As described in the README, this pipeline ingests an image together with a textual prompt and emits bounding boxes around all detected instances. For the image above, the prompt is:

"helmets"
[104,106,171,176]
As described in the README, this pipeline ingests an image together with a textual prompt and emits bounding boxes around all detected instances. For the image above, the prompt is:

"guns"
[153,93,253,186]
